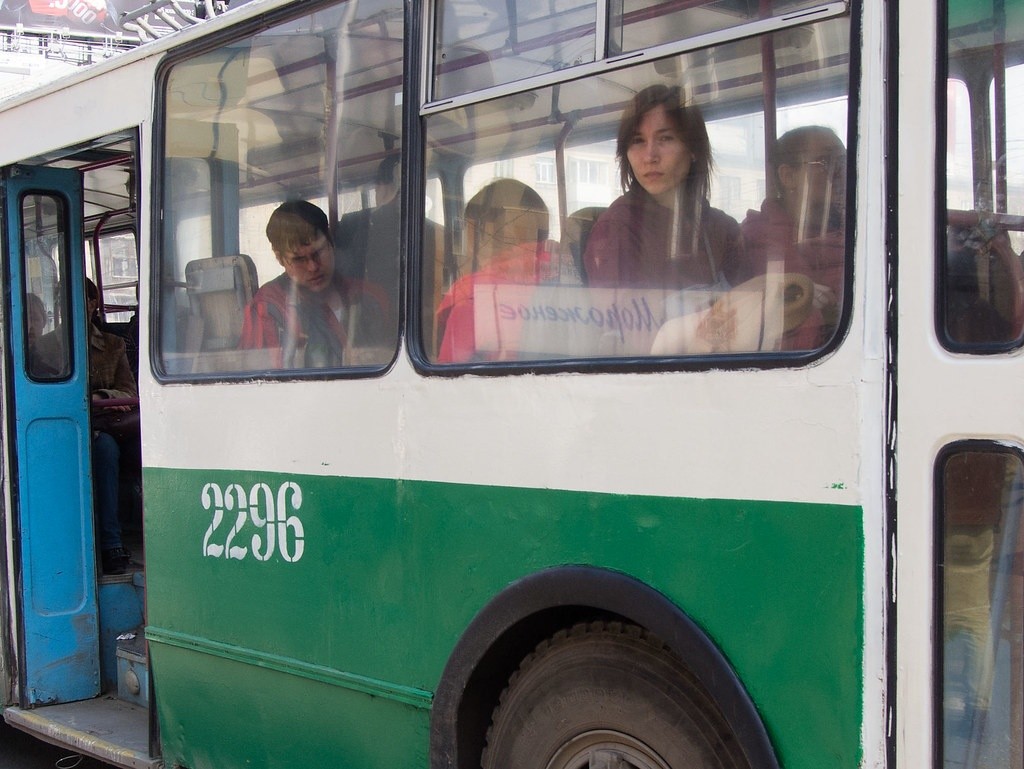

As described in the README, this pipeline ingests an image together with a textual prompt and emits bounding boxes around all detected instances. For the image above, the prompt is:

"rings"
[817,292,822,298]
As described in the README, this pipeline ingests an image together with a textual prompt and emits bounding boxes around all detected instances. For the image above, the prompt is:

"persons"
[26,293,47,349]
[29,277,141,574]
[583,84,837,355]
[125,271,175,384]
[335,149,445,366]
[241,199,395,368]
[723,126,847,342]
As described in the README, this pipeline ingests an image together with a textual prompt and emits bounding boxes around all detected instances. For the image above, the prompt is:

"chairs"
[186,254,261,352]
[561,207,607,286]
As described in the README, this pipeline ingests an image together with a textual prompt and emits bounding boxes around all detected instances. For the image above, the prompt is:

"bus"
[0,0,1024,769]
